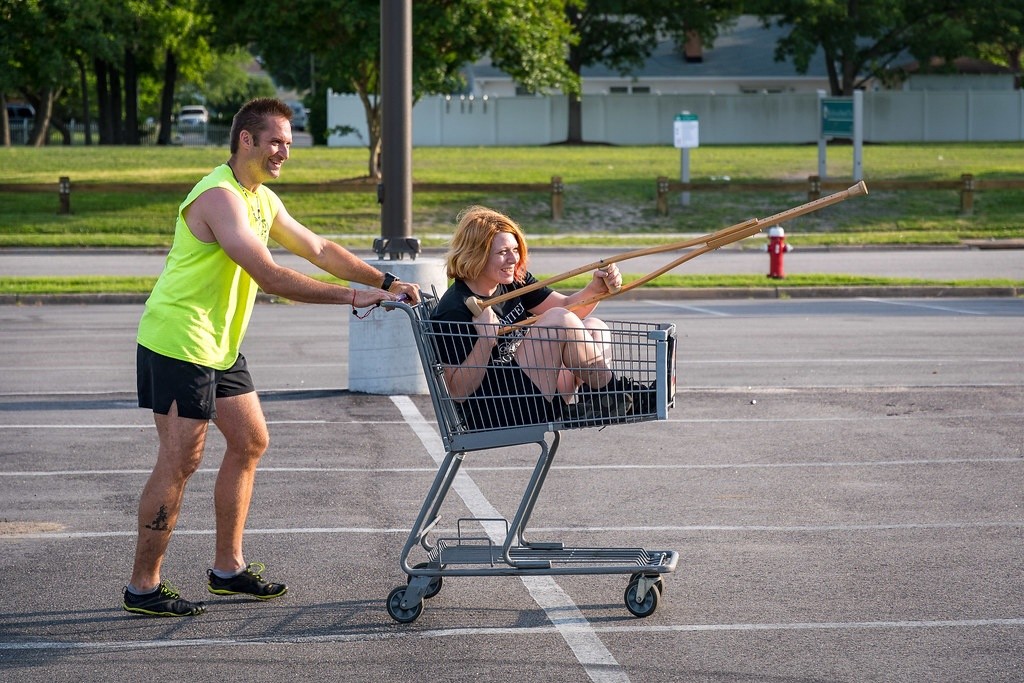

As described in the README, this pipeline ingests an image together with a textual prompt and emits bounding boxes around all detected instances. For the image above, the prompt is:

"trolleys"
[376,289,681,623]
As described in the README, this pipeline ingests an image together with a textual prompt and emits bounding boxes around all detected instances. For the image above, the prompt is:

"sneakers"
[207,562,289,599]
[564,377,648,426]
[122,579,206,616]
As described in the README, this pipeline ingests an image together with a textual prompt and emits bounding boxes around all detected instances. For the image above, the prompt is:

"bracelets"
[351,289,382,319]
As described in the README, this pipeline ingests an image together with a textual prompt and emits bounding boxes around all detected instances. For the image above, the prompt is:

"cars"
[176,105,209,126]
[6,103,36,122]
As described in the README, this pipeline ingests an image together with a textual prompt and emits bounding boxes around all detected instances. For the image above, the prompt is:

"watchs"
[381,272,400,289]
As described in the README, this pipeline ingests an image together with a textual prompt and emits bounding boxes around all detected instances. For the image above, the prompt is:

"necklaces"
[228,159,261,222]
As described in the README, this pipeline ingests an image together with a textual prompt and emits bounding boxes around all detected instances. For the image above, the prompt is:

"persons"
[122,97,421,616]
[429,206,658,429]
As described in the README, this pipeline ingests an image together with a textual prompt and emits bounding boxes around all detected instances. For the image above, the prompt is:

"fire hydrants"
[763,224,794,281]
